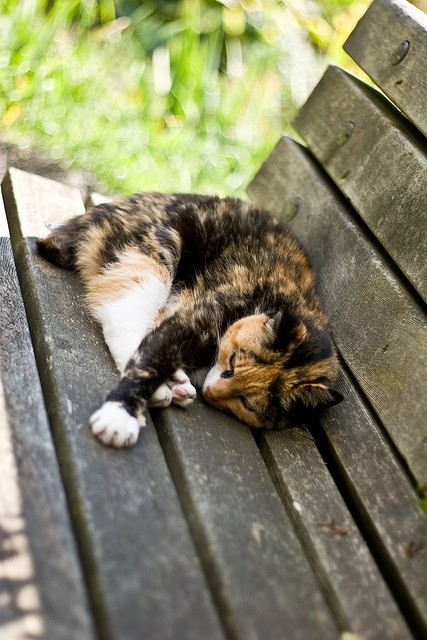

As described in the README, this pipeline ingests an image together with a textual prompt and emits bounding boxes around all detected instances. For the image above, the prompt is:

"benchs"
[1,0,426,636]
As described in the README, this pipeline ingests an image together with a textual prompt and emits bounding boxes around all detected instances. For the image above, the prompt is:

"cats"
[37,191,346,451]
